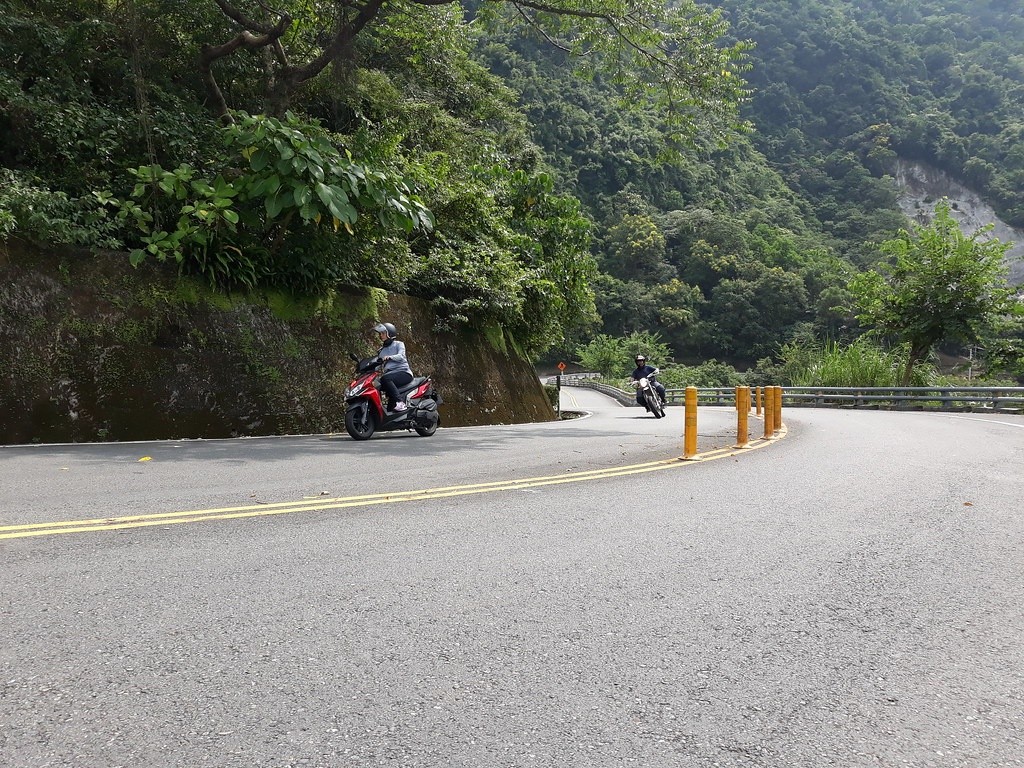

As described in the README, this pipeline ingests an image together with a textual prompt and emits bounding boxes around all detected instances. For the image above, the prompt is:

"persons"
[630,355,670,413]
[370,322,414,412]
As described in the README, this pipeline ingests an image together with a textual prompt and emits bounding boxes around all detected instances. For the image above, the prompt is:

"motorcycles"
[626,371,669,419]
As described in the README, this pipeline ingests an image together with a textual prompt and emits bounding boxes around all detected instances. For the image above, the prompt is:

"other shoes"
[645,406,650,412]
[662,399,667,403]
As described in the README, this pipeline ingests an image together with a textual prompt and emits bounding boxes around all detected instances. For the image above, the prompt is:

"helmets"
[372,323,396,339]
[635,355,645,364]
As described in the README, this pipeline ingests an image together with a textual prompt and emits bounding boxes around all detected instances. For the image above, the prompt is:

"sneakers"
[392,401,407,412]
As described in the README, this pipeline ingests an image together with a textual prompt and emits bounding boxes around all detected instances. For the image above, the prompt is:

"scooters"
[343,353,442,441]
